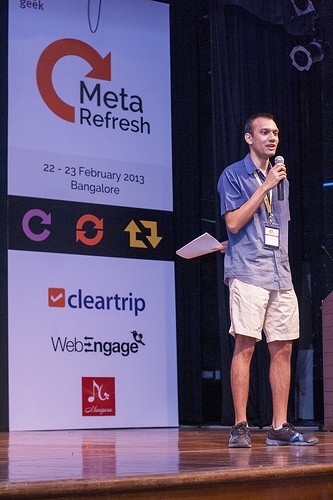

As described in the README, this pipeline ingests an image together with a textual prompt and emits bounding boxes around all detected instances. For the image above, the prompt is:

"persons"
[211,113,320,448]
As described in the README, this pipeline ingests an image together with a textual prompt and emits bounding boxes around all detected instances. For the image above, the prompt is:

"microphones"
[274,156,285,200]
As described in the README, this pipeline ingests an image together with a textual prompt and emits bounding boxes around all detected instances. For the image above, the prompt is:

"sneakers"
[266,423,319,445]
[228,422,252,447]
